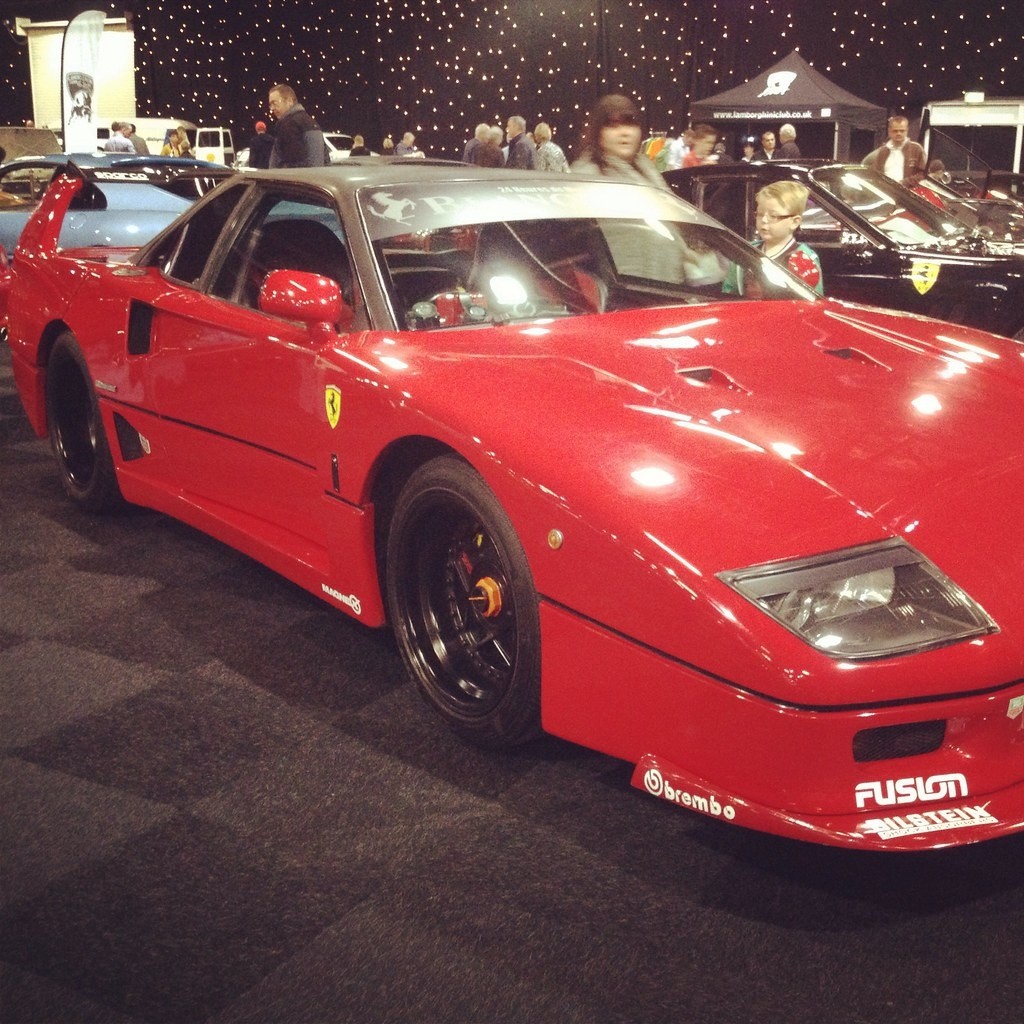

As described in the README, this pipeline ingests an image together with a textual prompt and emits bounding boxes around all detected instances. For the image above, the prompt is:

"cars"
[232,132,380,174]
[0,159,1024,857]
[660,158,1024,341]
[0,152,242,265]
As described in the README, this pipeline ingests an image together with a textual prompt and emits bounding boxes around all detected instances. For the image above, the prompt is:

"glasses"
[753,212,796,222]
[603,115,642,127]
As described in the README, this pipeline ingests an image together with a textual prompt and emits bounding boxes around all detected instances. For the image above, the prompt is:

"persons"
[722,181,823,298]
[462,123,506,168]
[160,126,195,160]
[349,135,370,156]
[877,115,926,188]
[249,122,275,169]
[928,159,945,182]
[381,138,394,155]
[502,116,572,173]
[665,119,800,174]
[393,132,416,155]
[26,119,34,127]
[104,121,151,155]
[269,83,330,168]
[568,94,708,285]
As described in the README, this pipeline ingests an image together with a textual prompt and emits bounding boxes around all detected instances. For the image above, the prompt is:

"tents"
[688,50,887,163]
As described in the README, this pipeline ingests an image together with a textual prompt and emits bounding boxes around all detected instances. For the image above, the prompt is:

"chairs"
[467,222,542,290]
[247,218,354,309]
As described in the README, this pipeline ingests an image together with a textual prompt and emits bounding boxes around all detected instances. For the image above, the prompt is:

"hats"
[256,122,266,130]
[930,160,944,172]
